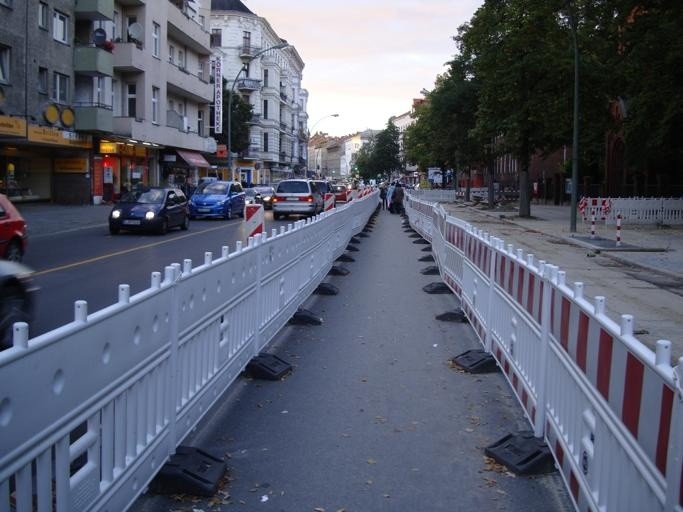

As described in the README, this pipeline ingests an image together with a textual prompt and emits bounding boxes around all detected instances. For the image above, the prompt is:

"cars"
[0,191,29,266]
[103,176,358,238]
[0,255,41,350]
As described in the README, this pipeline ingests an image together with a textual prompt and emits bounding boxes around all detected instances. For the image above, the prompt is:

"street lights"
[226,42,290,184]
[555,6,585,236]
[414,172,427,190]
[305,113,340,172]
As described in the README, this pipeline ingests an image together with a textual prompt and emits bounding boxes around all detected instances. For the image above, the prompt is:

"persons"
[379,178,404,215]
[241,176,245,184]
